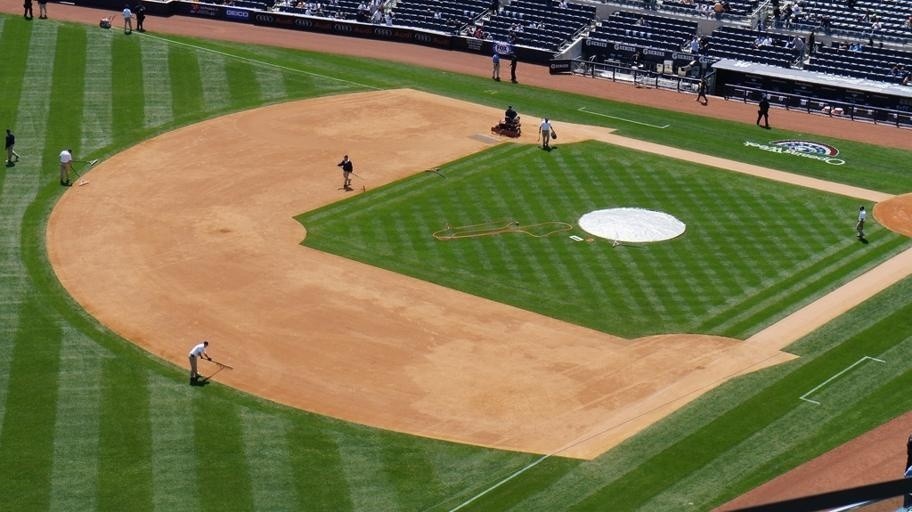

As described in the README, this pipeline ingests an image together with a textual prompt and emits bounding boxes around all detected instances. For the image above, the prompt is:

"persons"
[284,0,493,41]
[121,4,135,34]
[856,204,867,239]
[133,2,146,30]
[337,154,354,191]
[24,0,33,19]
[58,149,73,186]
[37,0,48,19]
[539,117,555,150]
[492,1,911,103]
[756,96,771,129]
[5,129,20,165]
[188,340,212,386]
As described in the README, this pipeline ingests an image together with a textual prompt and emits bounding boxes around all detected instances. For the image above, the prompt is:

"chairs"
[174,0,912,88]
